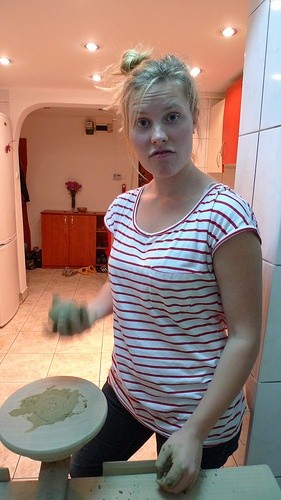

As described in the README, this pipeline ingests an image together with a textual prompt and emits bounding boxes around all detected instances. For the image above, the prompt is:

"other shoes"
[96,265,108,272]
[96,253,107,264]
[62,269,77,277]
[96,236,108,246]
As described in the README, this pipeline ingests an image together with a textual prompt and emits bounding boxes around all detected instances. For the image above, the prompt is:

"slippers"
[78,267,89,275]
[86,265,97,274]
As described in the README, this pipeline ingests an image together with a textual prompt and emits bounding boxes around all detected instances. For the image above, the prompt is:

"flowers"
[65,181,82,209]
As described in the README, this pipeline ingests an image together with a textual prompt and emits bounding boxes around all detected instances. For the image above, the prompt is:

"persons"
[52,49,263,495]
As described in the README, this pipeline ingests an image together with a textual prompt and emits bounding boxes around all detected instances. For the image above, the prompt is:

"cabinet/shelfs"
[40,209,112,269]
